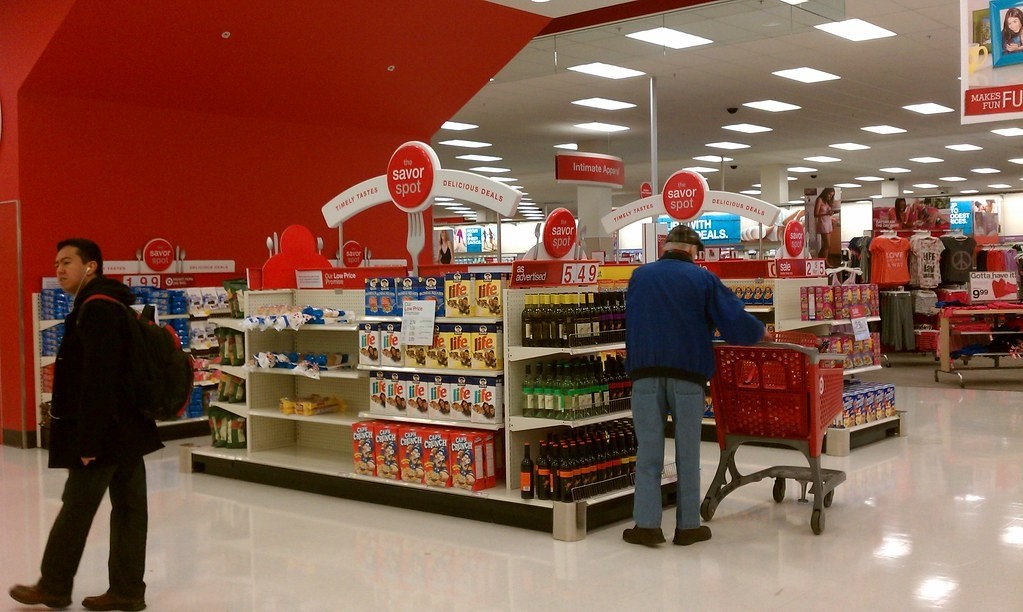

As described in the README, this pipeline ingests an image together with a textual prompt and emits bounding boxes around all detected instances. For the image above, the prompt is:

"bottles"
[520,443,534,499]
[535,421,636,503]
[522,291,626,347]
[522,354,631,420]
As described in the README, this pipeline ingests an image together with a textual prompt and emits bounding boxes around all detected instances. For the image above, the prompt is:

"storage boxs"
[396,276,419,316]
[359,322,380,365]
[506,271,521,290]
[428,374,450,421]
[378,278,397,316]
[419,276,445,317]
[404,345,426,367]
[39,285,227,420]
[368,370,387,414]
[405,373,429,419]
[700,283,896,428]
[915,330,939,351]
[449,375,471,421]
[470,377,503,424]
[380,322,406,366]
[364,277,379,315]
[424,324,448,369]
[444,271,475,317]
[352,418,505,494]
[384,370,407,416]
[470,324,503,370]
[447,323,472,369]
[475,271,506,317]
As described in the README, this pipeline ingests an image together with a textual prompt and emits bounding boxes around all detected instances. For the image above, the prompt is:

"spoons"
[579,225,588,260]
[266,236,274,259]
[180,250,185,274]
[368,250,372,266]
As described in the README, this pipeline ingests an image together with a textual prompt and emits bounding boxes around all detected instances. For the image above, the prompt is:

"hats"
[663,225,705,251]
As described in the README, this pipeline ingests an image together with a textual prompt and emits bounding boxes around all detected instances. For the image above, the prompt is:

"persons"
[437,230,454,264]
[814,188,841,268]
[482,228,496,250]
[975,200,994,212]
[372,393,495,420]
[887,198,950,230]
[622,225,772,545]
[458,297,500,316]
[361,346,497,368]
[10,238,164,612]
[1002,8,1023,54]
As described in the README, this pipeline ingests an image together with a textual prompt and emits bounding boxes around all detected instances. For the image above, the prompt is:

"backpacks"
[82,290,194,421]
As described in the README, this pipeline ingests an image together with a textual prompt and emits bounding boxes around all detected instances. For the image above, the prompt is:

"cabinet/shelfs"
[597,256,910,457]
[180,261,678,543]
[931,308,1023,390]
[32,294,231,456]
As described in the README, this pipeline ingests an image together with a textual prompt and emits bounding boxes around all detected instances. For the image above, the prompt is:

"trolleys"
[699,332,848,534]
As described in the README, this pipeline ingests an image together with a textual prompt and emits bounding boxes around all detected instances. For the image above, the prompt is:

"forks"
[336,249,340,267]
[317,237,324,256]
[533,223,542,261]
[135,249,142,274]
[407,213,425,279]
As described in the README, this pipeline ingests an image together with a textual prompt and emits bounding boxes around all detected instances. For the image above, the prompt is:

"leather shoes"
[9,579,72,608]
[81,581,146,612]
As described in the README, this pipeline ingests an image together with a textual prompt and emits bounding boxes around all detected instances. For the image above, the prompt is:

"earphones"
[86,267,91,272]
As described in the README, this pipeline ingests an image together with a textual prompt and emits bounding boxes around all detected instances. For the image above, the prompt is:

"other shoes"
[623,525,665,547]
[672,525,712,547]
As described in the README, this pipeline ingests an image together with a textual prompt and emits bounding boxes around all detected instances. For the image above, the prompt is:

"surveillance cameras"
[811,175,817,178]
[730,165,737,169]
[727,108,738,114]
[889,178,895,181]
[941,192,944,194]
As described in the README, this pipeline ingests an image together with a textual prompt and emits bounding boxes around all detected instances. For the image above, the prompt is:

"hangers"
[981,241,1023,259]
[912,230,937,240]
[824,261,863,278]
[941,228,968,239]
[878,230,901,239]
[881,286,937,297]
[939,284,968,295]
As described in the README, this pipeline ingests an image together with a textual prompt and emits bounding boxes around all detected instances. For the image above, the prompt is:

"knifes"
[364,247,368,268]
[574,218,581,260]
[175,246,180,273]
[273,232,278,255]
